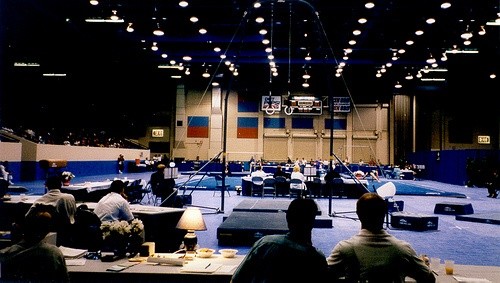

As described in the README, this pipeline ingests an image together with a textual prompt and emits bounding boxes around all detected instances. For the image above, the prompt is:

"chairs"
[275,175,287,198]
[71,208,111,253]
[331,178,344,199]
[250,177,264,198]
[289,178,304,199]
[213,175,231,197]
[263,177,277,200]
[139,181,151,204]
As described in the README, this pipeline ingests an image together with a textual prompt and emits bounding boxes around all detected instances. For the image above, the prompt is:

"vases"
[111,232,144,259]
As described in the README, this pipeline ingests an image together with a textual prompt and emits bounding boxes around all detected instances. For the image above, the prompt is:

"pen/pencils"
[205,263,211,269]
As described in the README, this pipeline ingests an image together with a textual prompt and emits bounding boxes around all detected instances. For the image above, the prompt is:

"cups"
[124,177,127,180]
[20,194,25,199]
[431,258,441,273]
[444,259,454,275]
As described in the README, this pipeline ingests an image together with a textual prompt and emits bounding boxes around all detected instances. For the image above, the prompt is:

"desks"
[0,160,500,283]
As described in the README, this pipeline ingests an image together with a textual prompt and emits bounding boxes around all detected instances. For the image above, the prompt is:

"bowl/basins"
[218,249,238,257]
[196,248,215,257]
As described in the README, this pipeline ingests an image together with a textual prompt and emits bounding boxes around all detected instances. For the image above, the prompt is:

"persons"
[467,157,500,197]
[287,156,323,165]
[25,174,76,225]
[230,198,328,283]
[145,153,169,165]
[151,164,178,197]
[324,164,341,181]
[0,161,14,185]
[118,154,124,173]
[290,166,308,191]
[0,210,71,283]
[273,164,288,179]
[370,169,381,183]
[343,157,382,166]
[251,166,267,186]
[0,179,25,231]
[326,193,437,283]
[92,180,135,225]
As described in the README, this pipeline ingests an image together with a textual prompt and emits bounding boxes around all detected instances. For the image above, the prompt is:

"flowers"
[100,217,145,241]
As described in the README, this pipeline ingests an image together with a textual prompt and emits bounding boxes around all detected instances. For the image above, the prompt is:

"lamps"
[175,206,207,252]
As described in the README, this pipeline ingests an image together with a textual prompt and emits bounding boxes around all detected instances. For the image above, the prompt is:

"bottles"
[424,254,429,265]
